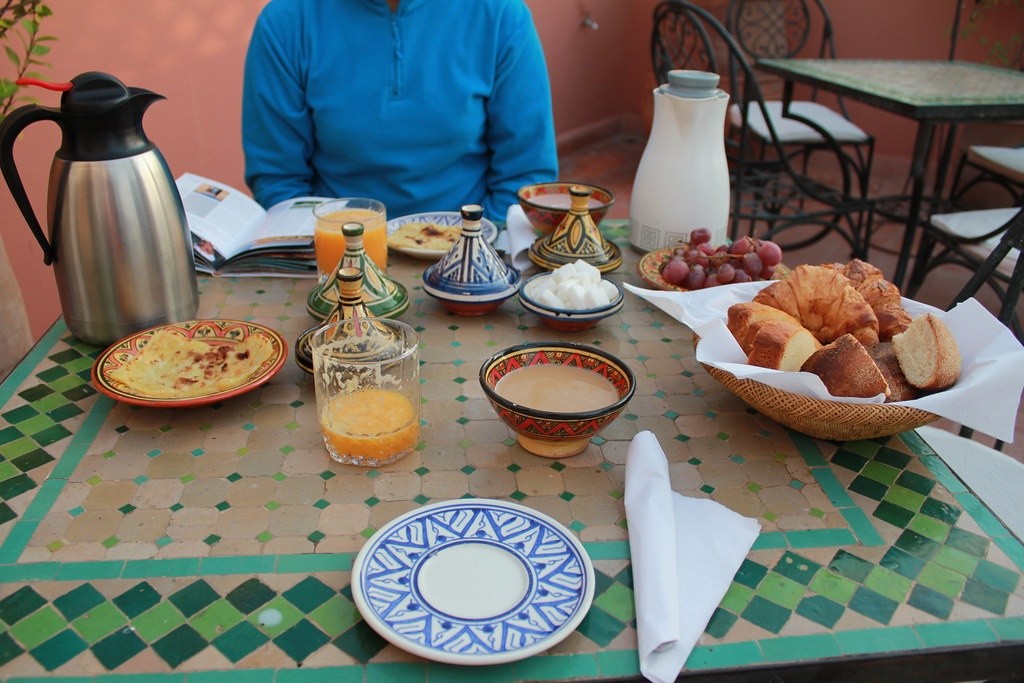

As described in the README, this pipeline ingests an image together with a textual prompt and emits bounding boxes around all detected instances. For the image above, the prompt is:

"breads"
[726,259,962,402]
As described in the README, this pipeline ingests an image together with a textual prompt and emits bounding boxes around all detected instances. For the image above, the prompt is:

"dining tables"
[0,219,1024,683]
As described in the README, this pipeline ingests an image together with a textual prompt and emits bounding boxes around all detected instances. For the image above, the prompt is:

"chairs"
[649,0,1024,452]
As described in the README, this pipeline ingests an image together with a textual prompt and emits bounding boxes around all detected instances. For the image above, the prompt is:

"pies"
[386,222,463,250]
[110,331,273,397]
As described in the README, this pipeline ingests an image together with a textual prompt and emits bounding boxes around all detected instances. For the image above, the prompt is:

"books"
[175,172,351,278]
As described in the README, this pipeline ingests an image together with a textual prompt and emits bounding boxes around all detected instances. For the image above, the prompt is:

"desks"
[752,61,1024,291]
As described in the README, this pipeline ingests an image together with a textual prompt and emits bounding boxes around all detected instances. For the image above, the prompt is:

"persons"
[241,0,559,224]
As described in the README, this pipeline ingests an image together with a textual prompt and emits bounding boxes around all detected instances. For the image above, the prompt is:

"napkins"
[623,431,761,683]
[506,204,539,268]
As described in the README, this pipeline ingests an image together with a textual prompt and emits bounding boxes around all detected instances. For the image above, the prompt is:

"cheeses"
[531,259,618,309]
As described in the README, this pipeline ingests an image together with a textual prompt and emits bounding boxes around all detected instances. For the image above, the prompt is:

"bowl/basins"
[422,264,521,315]
[516,181,616,236]
[479,341,636,459]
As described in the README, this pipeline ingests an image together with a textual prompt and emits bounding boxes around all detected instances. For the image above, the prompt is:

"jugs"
[0,72,200,348]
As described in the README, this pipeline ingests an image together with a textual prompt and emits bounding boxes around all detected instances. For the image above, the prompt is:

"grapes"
[662,228,781,291]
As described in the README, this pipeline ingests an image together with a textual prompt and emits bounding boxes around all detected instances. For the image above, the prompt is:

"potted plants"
[0,0,59,382]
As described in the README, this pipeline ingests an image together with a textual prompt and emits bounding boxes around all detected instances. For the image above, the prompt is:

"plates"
[636,247,795,298]
[90,322,287,409]
[349,497,595,665]
[386,209,498,259]
[515,269,625,330]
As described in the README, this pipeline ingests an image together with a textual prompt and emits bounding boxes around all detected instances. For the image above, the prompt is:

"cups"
[313,197,388,277]
[307,318,422,467]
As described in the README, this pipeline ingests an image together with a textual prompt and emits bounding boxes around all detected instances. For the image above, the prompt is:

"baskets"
[690,330,944,441]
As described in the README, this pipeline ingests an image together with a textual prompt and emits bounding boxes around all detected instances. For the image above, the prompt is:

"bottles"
[625,67,732,257]
